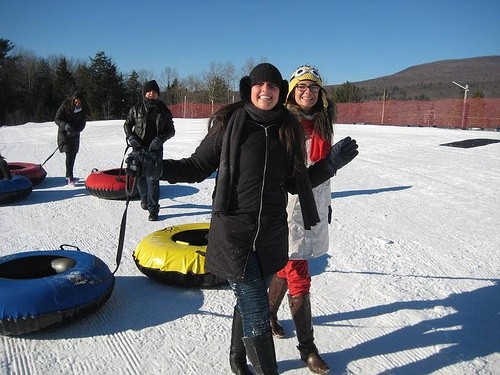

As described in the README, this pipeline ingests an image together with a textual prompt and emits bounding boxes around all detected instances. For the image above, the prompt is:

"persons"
[269,66,338,375]
[124,62,359,375]
[123,80,175,221]
[54,92,91,186]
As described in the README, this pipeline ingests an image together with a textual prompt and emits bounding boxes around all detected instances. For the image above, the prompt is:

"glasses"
[295,83,321,93]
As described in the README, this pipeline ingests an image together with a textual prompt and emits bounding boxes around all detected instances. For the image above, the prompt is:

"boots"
[242,327,278,375]
[268,276,289,339]
[287,292,330,375]
[230,306,255,375]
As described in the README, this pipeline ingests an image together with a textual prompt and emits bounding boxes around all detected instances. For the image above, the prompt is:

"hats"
[284,65,328,108]
[249,62,282,88]
[143,80,160,96]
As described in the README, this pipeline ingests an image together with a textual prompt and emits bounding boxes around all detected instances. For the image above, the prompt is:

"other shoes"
[141,199,148,209]
[67,177,76,187]
[148,212,158,221]
[65,177,78,184]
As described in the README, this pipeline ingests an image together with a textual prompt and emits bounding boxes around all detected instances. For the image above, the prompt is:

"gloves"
[325,136,358,175]
[65,123,73,132]
[125,152,163,180]
[68,130,78,137]
[148,137,163,151]
[128,134,144,148]
